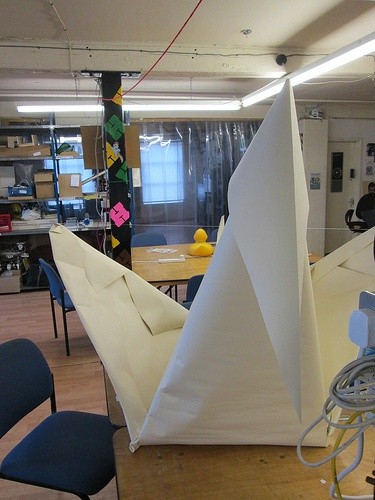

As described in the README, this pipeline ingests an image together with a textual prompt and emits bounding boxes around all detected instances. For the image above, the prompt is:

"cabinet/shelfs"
[0,125,135,290]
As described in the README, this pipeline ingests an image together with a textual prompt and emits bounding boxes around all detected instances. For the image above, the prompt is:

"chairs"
[38,258,76,355]
[180,275,204,311]
[131,233,178,303]
[0,338,121,500]
[345,193,375,233]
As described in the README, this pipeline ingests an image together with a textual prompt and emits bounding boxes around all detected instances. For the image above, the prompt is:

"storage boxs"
[0,144,52,158]
[34,169,55,198]
[59,173,82,197]
[0,270,21,293]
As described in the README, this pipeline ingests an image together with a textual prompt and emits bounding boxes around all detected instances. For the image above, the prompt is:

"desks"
[129,243,324,295]
[102,364,375,499]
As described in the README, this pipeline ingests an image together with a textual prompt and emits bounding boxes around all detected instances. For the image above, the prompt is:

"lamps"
[240,32,375,109]
[13,76,241,112]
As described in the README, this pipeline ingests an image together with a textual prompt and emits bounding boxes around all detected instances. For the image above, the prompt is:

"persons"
[355,183,375,227]
